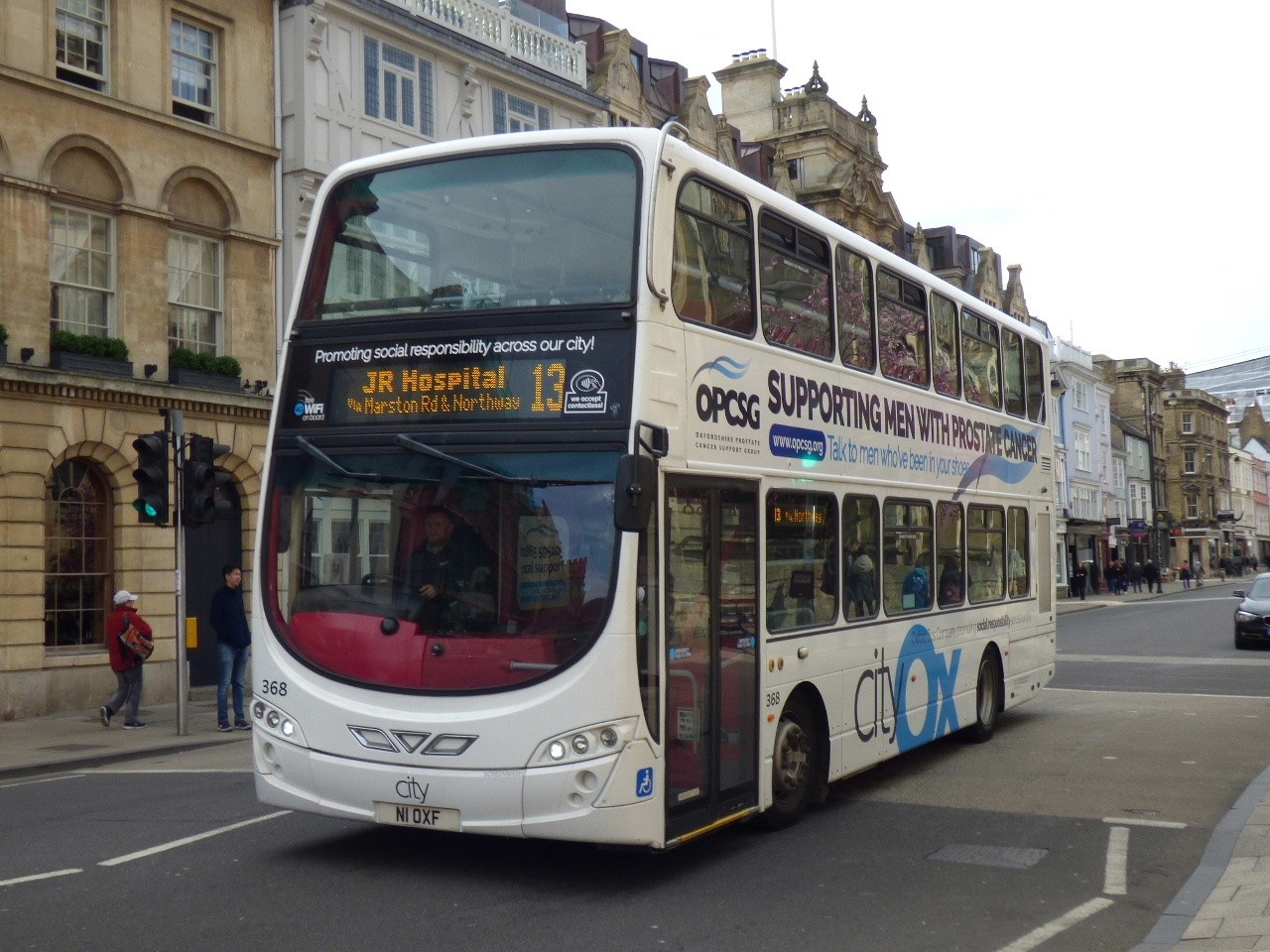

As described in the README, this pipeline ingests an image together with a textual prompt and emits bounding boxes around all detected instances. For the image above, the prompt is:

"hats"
[113,590,138,605]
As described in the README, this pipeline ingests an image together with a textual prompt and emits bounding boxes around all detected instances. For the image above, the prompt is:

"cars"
[1233,572,1270,651]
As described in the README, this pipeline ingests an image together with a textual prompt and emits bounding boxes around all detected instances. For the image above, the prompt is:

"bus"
[249,120,1059,853]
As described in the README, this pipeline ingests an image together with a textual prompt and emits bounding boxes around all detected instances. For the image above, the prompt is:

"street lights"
[1148,384,1177,594]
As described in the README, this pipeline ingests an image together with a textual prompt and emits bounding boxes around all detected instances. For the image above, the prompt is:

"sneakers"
[216,722,233,732]
[235,720,252,730]
[123,720,146,729]
[100,705,112,727]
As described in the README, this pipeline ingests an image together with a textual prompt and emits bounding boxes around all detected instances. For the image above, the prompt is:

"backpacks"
[942,582,959,604]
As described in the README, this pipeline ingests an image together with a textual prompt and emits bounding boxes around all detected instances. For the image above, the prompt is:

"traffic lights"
[191,437,234,522]
[132,432,169,524]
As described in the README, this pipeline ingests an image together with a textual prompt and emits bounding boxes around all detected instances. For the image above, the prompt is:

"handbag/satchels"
[120,612,154,660]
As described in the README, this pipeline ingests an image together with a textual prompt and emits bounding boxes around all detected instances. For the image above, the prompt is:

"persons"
[98,590,148,728]
[1131,562,1142,593]
[1211,554,1259,578]
[1180,559,1191,588]
[1075,563,1088,600]
[1266,553,1270,572]
[209,564,253,731]
[1194,561,1207,588]
[831,539,875,621]
[938,556,974,606]
[1090,558,1128,596]
[394,508,486,625]
[975,543,1026,601]
[901,554,931,610]
[1142,559,1156,593]
